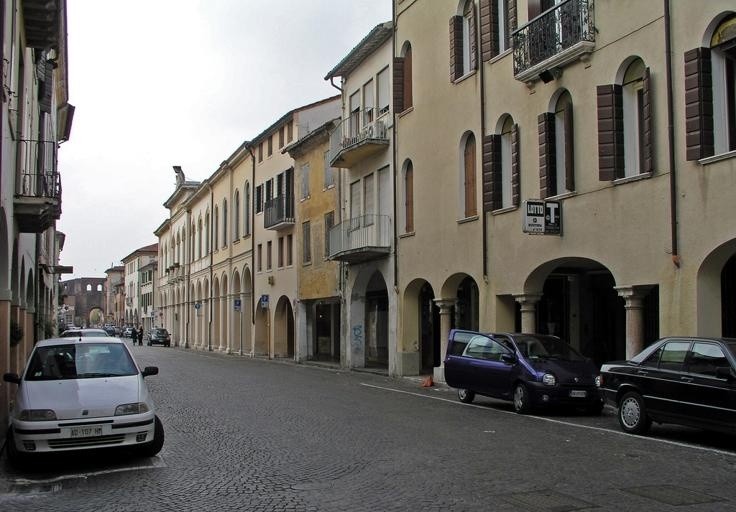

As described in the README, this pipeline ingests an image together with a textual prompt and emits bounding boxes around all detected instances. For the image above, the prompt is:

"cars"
[597,336,736,446]
[443,326,604,420]
[4,321,172,468]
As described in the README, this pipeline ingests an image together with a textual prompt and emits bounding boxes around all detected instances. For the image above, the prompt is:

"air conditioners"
[368,120,385,139]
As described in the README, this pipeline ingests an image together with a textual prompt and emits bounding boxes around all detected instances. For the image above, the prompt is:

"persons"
[137,326,143,346]
[131,327,138,345]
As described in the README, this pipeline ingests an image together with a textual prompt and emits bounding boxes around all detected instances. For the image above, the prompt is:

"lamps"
[539,67,563,83]
